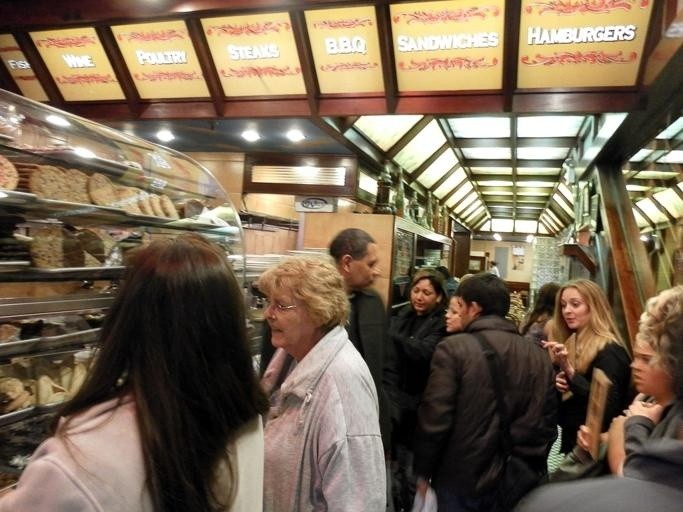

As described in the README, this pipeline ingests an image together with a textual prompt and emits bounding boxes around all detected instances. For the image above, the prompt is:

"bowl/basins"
[250,307,265,318]
[424,261,430,265]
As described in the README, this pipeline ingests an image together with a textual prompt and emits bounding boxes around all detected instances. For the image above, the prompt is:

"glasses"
[271,304,296,313]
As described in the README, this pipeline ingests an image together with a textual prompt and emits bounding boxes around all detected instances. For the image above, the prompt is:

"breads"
[0,307,111,341]
[29,225,122,268]
[0,352,99,419]
[0,144,203,218]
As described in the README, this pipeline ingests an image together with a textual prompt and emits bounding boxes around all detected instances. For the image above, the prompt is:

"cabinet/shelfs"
[295,211,456,308]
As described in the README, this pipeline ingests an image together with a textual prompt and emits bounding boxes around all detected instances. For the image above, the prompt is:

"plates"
[250,317,265,323]
[226,247,329,273]
[421,265,437,267]
[424,249,442,260]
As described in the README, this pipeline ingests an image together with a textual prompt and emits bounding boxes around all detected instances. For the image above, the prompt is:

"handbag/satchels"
[499,451,548,508]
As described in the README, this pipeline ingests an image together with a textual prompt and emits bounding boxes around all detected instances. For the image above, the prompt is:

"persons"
[259,228,400,512]
[389,260,683,512]
[0,230,270,512]
[251,258,387,512]
